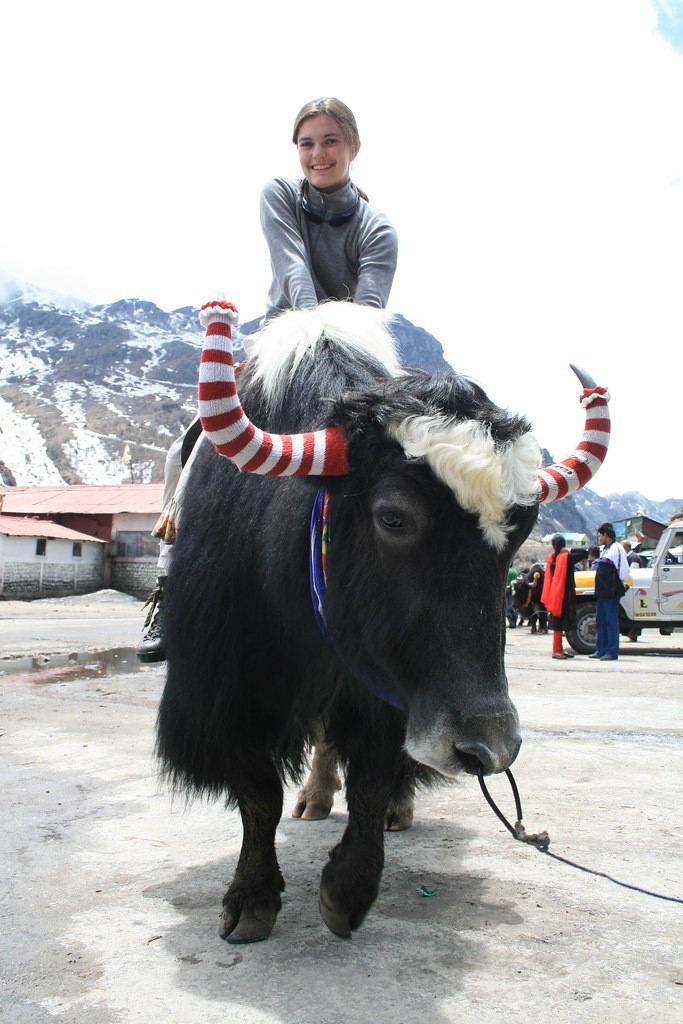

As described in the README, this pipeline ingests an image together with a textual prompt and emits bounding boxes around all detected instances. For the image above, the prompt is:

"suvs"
[563,522,682,655]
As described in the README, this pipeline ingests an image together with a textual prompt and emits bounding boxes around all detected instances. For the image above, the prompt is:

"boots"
[507,616,516,628]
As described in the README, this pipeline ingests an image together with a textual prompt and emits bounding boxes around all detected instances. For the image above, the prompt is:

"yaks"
[149,296,613,948]
[506,557,550,635]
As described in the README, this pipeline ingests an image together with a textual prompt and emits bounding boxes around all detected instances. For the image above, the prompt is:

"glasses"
[300,179,360,227]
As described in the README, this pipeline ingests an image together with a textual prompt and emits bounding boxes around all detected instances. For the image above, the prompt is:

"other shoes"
[552,653,567,659]
[600,655,613,660]
[563,652,574,658]
[589,654,598,658]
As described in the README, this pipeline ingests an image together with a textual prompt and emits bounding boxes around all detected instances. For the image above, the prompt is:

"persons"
[137,96,398,663]
[540,523,641,661]
[506,561,518,628]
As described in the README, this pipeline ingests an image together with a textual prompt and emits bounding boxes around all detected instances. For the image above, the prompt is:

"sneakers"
[136,576,167,662]
